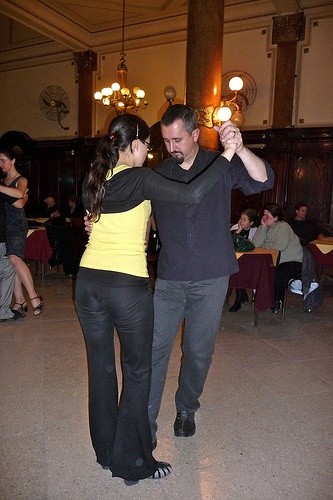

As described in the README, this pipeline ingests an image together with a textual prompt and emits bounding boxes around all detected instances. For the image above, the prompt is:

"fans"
[39,86,71,130]
[220,70,257,113]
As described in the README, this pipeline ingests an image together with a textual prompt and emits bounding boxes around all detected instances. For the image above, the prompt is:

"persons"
[0,144,45,320]
[36,192,89,278]
[84,104,278,448]
[229,203,326,312]
[72,114,238,488]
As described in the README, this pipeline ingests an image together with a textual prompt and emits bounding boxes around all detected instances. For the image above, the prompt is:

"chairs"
[282,246,324,316]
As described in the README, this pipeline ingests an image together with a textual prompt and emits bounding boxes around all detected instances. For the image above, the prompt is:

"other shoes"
[240,288,248,304]
[228,300,242,313]
[271,300,283,314]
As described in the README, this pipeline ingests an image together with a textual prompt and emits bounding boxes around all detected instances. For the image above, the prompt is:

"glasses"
[142,140,154,152]
[239,218,249,223]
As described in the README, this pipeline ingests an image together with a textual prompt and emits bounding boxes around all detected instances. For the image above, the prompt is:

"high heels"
[124,461,172,486]
[13,299,28,313]
[30,293,43,316]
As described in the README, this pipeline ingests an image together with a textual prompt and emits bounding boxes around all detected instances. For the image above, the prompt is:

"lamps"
[164,87,177,108]
[212,77,245,127]
[94,0,149,115]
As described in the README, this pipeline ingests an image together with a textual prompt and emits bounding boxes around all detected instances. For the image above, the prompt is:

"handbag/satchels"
[234,233,255,251]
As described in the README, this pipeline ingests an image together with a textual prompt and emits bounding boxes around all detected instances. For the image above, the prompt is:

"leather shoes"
[151,438,157,451]
[0,312,26,322]
[174,413,195,437]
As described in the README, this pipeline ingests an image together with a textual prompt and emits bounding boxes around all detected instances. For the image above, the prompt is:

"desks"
[308,238,333,288]
[24,227,53,282]
[146,246,280,327]
